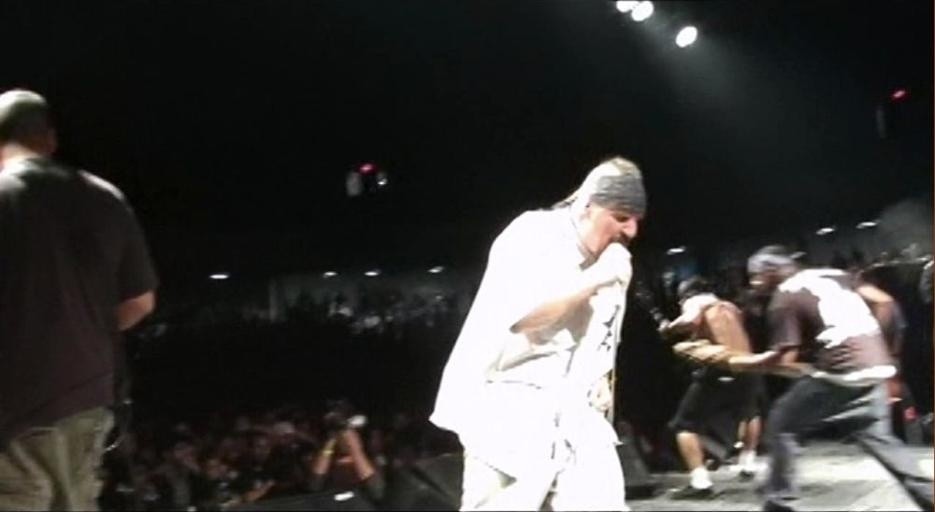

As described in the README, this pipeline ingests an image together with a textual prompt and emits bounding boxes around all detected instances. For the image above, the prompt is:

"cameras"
[323,414,348,433]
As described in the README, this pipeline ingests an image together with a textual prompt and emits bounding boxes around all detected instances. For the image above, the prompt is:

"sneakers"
[665,467,759,501]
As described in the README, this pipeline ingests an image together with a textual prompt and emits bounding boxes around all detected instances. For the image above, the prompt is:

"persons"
[0,89,163,510]
[612,243,935,512]
[422,154,653,510]
[95,275,466,511]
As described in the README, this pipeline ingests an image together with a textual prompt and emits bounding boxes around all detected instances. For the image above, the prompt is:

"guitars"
[632,280,683,346]
[675,340,812,381]
